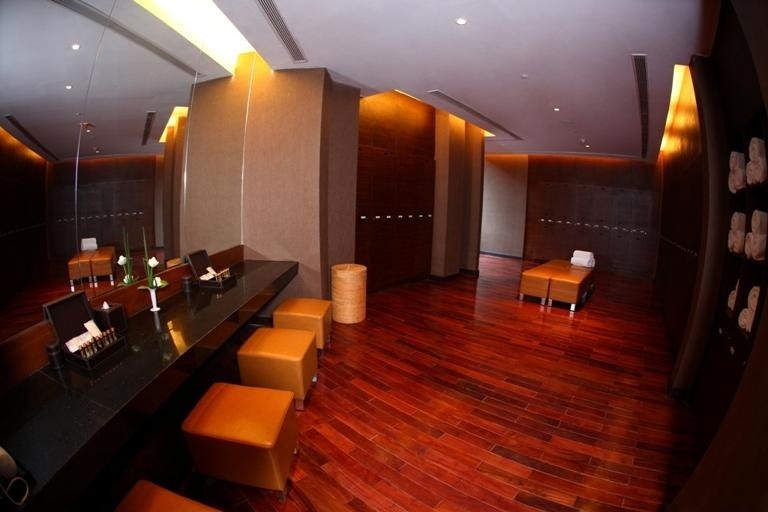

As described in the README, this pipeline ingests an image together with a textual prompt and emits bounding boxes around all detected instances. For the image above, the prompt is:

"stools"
[238,328,317,410]
[182,383,299,503]
[274,297,332,354]
[111,480,221,512]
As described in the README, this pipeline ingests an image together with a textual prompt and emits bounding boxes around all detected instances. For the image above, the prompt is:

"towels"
[724,142,768,334]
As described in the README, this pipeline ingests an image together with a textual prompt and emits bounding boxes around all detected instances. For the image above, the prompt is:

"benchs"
[92,246,117,287]
[68,249,92,285]
[548,265,595,311]
[519,259,570,306]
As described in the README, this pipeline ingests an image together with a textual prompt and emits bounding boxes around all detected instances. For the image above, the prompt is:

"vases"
[149,289,160,311]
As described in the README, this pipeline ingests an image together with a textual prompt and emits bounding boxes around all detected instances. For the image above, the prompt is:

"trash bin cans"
[331,264,367,324]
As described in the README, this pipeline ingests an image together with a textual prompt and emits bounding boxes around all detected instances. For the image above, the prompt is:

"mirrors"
[1,1,197,342]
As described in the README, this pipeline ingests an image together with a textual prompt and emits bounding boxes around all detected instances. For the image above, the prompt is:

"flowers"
[144,257,168,288]
[117,256,139,287]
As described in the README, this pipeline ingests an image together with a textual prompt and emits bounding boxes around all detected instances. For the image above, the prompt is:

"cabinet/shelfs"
[687,181,768,449]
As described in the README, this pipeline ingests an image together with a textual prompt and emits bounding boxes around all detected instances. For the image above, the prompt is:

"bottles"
[181,273,194,293]
[45,339,64,370]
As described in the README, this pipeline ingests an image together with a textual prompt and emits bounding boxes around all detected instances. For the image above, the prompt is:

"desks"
[1,258,298,511]
